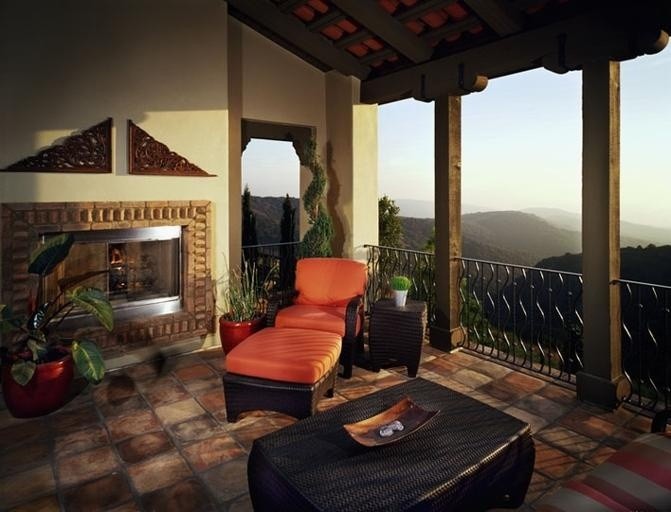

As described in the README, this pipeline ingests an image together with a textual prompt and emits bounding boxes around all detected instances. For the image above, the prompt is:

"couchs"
[535,407,671,512]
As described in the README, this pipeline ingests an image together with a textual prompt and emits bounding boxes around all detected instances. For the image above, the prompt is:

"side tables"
[368,298,428,378]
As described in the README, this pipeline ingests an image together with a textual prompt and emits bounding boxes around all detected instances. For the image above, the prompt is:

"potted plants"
[390,275,413,307]
[209,248,268,357]
[0,234,115,419]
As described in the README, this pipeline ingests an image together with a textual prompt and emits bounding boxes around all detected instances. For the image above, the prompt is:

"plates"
[344,397,441,448]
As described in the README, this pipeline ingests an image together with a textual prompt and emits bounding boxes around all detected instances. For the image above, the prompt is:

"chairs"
[274,257,368,379]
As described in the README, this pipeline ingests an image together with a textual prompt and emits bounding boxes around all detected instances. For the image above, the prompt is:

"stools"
[221,327,342,421]
